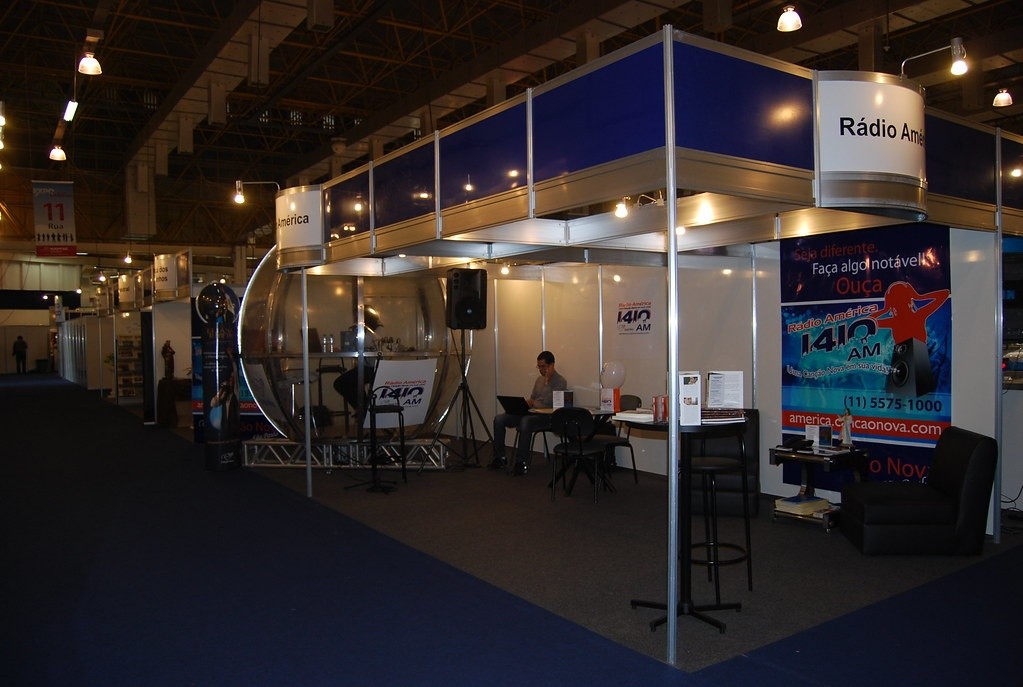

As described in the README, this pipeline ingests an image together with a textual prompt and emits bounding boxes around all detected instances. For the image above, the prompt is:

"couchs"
[832,424,998,557]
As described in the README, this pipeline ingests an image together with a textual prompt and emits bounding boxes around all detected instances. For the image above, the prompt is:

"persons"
[210,376,238,435]
[334,315,376,415]
[487,350,567,476]
[686,398,693,404]
[840,407,852,444]
[12,336,28,376]
[688,376,697,383]
[161,339,175,377]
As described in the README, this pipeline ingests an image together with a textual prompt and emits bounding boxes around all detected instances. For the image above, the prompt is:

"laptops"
[496,394,534,416]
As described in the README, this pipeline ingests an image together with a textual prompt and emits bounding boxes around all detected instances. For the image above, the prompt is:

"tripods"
[416,329,510,478]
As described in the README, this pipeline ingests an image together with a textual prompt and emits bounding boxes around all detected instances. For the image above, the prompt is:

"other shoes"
[488,456,509,469]
[509,463,529,476]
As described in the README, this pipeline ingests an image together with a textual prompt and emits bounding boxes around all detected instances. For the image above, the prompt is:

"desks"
[528,406,617,438]
[774,443,865,531]
[611,411,753,635]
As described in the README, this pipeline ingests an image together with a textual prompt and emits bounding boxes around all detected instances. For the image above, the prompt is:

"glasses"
[536,364,550,370]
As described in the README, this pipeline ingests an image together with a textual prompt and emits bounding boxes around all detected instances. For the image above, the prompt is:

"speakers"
[444,269,487,330]
[887,338,932,398]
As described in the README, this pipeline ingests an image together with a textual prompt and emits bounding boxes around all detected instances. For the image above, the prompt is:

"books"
[610,410,654,422]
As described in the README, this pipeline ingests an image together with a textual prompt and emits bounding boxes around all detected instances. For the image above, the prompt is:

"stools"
[677,407,759,601]
[370,402,407,493]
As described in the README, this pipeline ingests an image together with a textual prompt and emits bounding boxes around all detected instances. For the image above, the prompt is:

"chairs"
[507,392,575,474]
[596,394,641,486]
[546,406,609,505]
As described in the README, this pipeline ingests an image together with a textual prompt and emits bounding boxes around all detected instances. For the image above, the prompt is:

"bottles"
[321,334,327,353]
[329,334,335,353]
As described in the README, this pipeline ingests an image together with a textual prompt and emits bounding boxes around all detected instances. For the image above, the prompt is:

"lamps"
[900,36,969,77]
[235,180,280,204]
